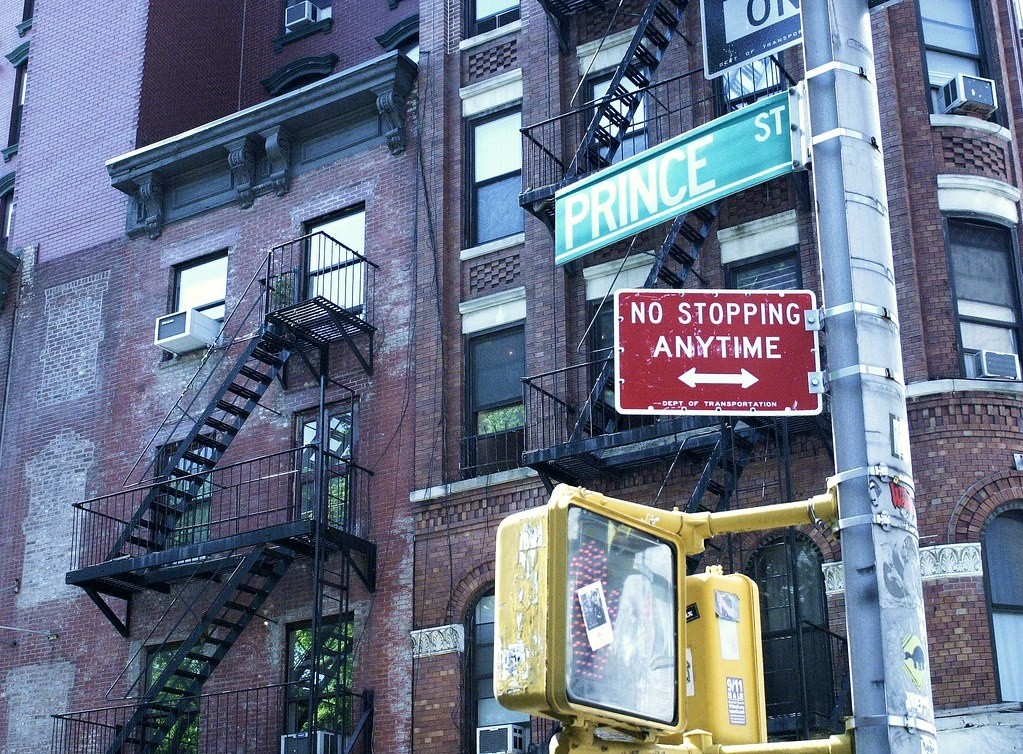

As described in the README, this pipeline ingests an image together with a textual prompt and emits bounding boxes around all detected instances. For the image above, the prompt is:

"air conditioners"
[944,74,998,119]
[476,724,526,754]
[153,309,221,355]
[282,731,342,754]
[973,350,1023,381]
[284,0,318,31]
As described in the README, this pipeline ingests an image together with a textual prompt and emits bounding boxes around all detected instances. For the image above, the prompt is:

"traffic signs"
[615,288,822,417]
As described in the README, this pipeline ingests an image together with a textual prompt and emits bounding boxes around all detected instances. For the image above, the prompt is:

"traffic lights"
[493,494,688,753]
